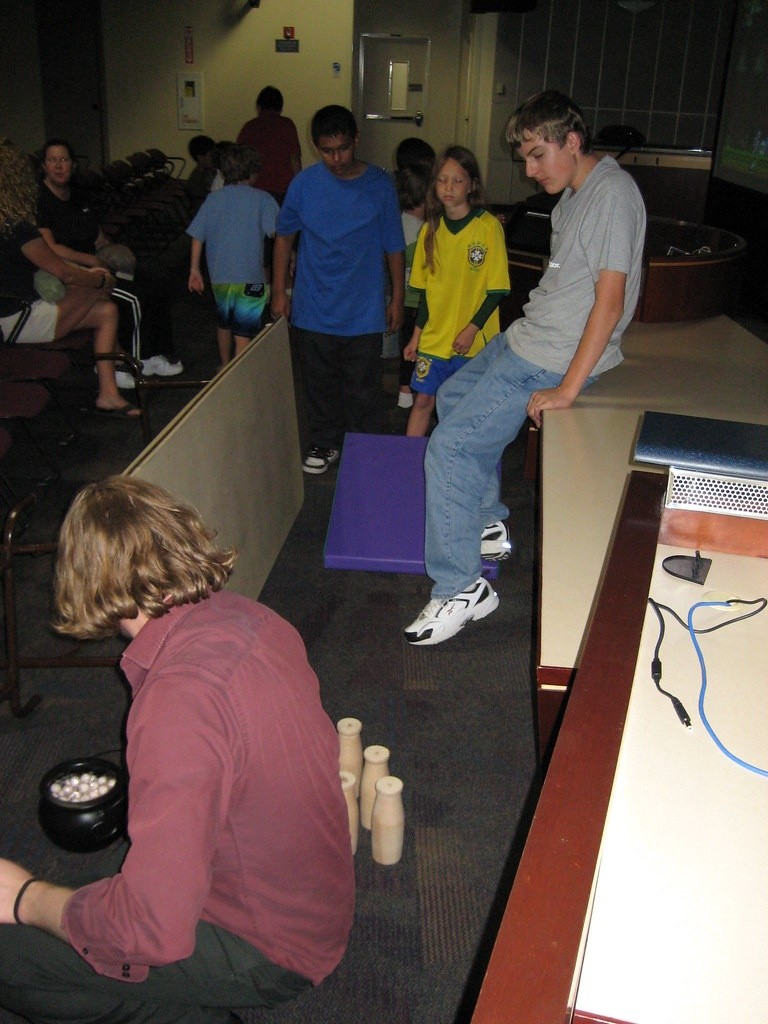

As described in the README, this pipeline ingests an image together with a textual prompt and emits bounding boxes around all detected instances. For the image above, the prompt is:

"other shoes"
[397,392,415,408]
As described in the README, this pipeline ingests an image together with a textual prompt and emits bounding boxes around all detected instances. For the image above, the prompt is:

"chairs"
[0,147,194,518]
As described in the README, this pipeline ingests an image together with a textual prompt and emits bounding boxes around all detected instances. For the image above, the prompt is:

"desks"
[471,314,767,1024]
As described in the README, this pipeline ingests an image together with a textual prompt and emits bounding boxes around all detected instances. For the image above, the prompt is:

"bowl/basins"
[38,757,130,854]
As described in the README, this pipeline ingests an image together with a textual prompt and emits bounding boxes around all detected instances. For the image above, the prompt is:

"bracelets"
[14,877,42,924]
[96,272,105,288]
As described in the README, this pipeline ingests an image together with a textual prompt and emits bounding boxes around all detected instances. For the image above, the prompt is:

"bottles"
[336,718,405,865]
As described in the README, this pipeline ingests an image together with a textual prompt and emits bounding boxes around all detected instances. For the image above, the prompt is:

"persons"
[0,138,154,420]
[0,476,356,1024]
[395,133,437,408]
[270,104,406,469]
[402,141,509,437]
[403,87,650,650]
[34,139,183,389]
[183,86,304,374]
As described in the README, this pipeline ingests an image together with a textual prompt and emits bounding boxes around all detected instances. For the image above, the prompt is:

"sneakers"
[402,576,500,646]
[140,355,183,376]
[480,519,511,562]
[302,444,339,475]
[94,361,136,389]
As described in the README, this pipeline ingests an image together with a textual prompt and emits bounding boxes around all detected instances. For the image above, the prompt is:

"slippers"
[93,404,141,421]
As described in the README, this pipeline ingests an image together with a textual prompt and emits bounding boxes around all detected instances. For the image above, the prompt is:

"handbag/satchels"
[597,125,646,160]
[32,268,65,305]
